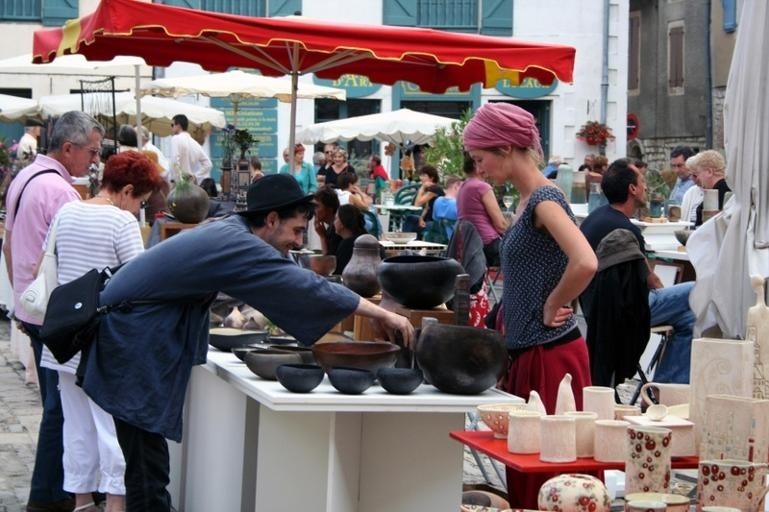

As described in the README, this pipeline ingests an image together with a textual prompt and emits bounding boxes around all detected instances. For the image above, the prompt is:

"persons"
[579,152,608,194]
[577,156,700,387]
[245,140,511,278]
[2,111,212,512]
[73,173,417,512]
[669,146,732,226]
[462,101,604,510]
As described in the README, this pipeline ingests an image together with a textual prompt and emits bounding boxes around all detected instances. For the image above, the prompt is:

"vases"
[221,158,233,167]
[166,174,211,224]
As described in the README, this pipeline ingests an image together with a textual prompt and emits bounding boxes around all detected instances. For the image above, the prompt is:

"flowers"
[576,120,615,145]
[218,123,235,157]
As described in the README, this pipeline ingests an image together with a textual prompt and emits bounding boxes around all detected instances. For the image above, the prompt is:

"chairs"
[360,153,768,405]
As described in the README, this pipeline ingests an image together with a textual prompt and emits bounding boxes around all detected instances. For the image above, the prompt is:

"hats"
[233,173,314,218]
[594,228,645,274]
[25,117,45,127]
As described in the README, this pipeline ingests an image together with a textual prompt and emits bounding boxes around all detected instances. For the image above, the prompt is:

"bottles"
[668,200,682,222]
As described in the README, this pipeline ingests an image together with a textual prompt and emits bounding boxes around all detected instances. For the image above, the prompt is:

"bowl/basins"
[673,230,695,246]
[231,336,424,395]
[475,403,527,439]
[385,231,417,244]
[208,328,271,353]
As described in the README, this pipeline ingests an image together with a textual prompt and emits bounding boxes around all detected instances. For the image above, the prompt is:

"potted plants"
[233,129,260,169]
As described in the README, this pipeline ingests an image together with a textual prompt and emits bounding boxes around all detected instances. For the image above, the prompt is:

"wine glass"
[503,196,514,213]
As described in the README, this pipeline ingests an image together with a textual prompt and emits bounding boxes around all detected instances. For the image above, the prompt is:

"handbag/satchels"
[19,251,104,365]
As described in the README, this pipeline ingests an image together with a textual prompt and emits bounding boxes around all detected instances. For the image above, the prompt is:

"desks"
[449,430,699,509]
[157,218,196,242]
[164,342,525,512]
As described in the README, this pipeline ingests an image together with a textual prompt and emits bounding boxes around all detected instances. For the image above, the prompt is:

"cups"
[624,491,746,512]
[649,199,665,218]
[696,459,769,512]
[624,425,674,495]
[581,385,616,419]
[614,404,643,423]
[539,414,577,464]
[506,409,541,455]
[593,420,630,464]
[563,411,600,460]
